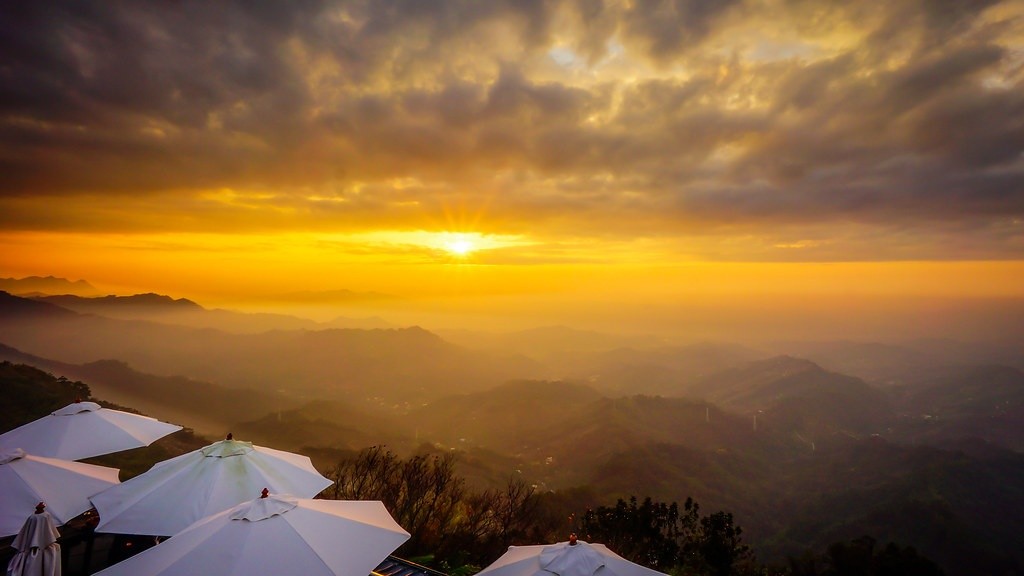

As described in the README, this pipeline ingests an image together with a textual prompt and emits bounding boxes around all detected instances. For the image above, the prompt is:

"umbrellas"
[0,448,120,537]
[88,433,334,536]
[6,502,61,576]
[473,533,669,576]
[92,487,411,576]
[0,398,183,460]
